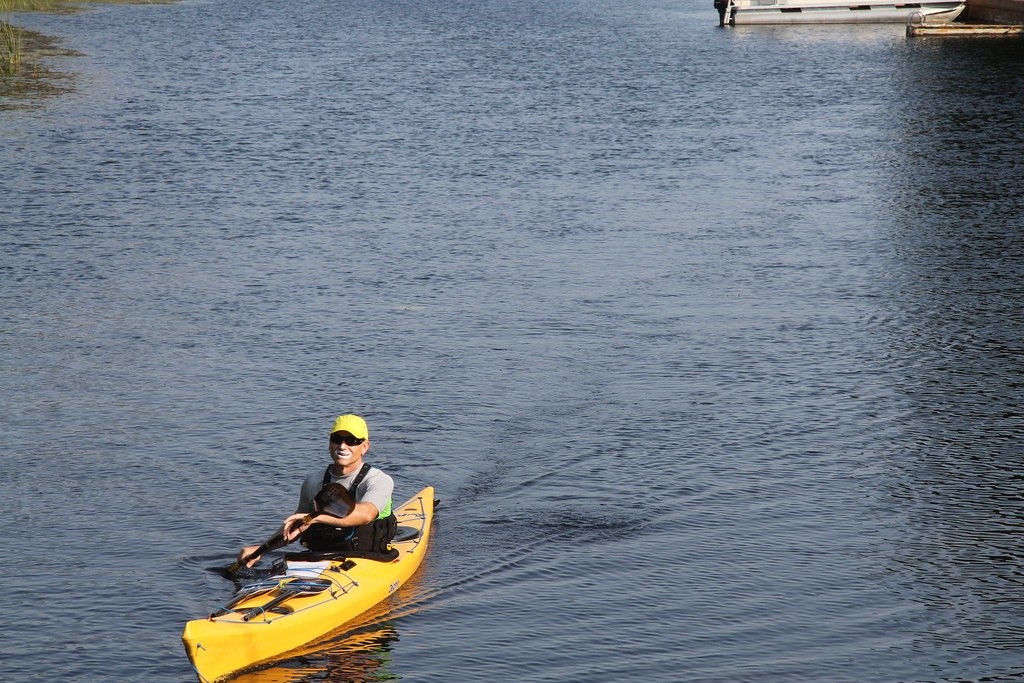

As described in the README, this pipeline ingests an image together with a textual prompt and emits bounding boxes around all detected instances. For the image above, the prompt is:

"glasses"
[330,434,364,446]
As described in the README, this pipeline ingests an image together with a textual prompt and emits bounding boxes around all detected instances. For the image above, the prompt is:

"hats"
[329,414,368,440]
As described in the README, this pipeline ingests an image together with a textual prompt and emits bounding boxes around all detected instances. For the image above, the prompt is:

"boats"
[713,0,966,26]
[180,486,434,683]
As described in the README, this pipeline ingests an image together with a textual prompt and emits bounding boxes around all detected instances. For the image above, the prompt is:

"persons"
[237,414,394,570]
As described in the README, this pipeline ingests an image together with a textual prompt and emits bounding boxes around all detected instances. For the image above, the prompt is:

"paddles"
[231,509,323,571]
[210,578,281,618]
[243,579,331,624]
